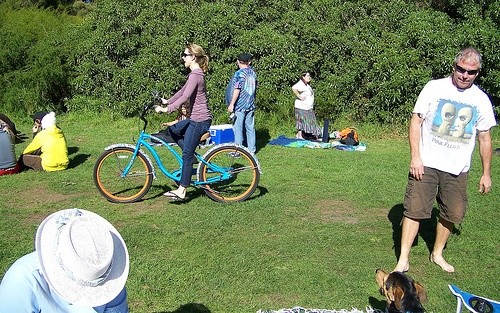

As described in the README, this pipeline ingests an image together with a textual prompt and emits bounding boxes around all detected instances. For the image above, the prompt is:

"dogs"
[374,268,427,313]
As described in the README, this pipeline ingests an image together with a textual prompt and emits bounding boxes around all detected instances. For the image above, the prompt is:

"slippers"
[193,162,199,164]
[163,191,189,201]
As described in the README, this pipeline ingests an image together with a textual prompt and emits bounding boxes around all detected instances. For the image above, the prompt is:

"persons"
[0,113,18,144]
[224,53,258,158]
[19,111,70,172]
[292,71,319,140]
[1,208,131,313]
[389,47,497,274]
[162,103,212,146]
[0,121,21,176]
[31,111,61,155]
[154,43,212,200]
[333,128,359,146]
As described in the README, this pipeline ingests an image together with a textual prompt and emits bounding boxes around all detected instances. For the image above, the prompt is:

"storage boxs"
[207,123,235,145]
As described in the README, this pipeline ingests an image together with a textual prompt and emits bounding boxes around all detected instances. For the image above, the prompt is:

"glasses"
[455,65,480,76]
[183,53,192,57]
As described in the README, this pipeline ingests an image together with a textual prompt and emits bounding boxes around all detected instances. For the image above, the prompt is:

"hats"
[42,112,57,128]
[31,111,46,120]
[35,208,130,308]
[238,53,253,62]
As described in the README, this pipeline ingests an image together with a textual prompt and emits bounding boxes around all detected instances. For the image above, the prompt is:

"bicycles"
[93,92,263,204]
